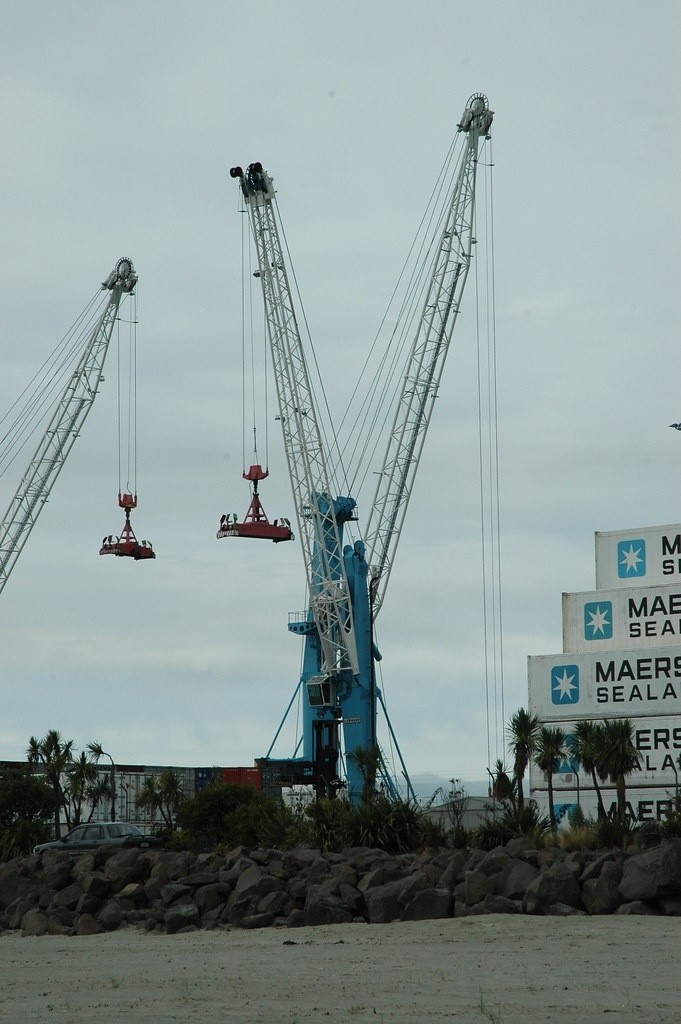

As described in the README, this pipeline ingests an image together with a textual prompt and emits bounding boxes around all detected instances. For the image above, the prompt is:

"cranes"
[212,90,498,829]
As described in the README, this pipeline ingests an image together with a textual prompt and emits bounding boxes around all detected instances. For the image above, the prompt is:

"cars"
[33,821,156,860]
